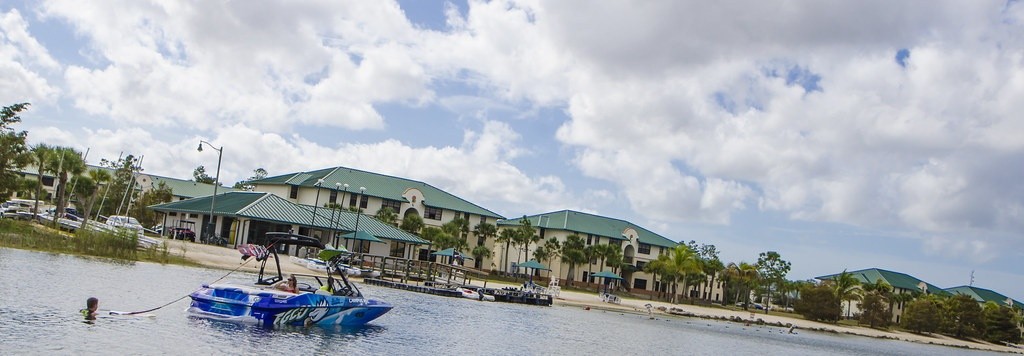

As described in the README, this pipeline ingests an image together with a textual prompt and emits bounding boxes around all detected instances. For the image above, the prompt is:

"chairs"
[599,292,622,305]
[521,280,561,298]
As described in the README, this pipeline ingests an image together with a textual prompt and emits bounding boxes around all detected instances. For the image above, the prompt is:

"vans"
[105,215,144,235]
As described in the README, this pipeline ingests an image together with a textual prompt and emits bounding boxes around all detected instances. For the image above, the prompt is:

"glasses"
[288,278,294,281]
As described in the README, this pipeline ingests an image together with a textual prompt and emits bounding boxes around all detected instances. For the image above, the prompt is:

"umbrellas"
[336,227,387,259]
[589,270,623,294]
[428,246,475,267]
[512,258,552,285]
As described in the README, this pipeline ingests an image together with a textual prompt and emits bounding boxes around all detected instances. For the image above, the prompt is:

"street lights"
[197,140,223,243]
[331,183,348,245]
[326,182,342,244]
[349,186,367,266]
[307,178,324,236]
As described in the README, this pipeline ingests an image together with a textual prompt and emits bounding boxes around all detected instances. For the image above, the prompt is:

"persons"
[318,276,336,295]
[271,274,300,292]
[80,297,99,316]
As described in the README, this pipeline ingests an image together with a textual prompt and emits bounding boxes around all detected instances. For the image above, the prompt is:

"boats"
[289,256,381,278]
[184,230,394,330]
[457,286,495,302]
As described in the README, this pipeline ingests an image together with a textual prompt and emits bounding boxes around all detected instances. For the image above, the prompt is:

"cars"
[169,228,196,242]
